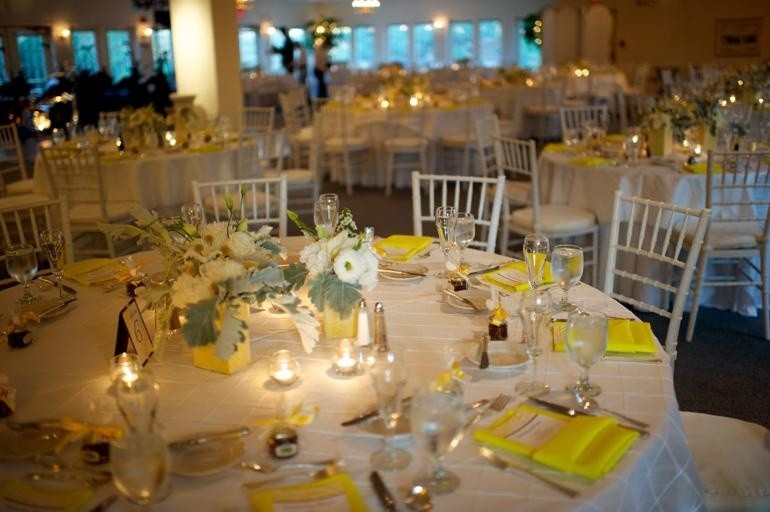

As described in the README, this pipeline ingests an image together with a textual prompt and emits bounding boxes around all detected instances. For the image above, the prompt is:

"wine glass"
[40,231,66,300]
[454,212,476,275]
[324,85,770,174]
[51,112,230,155]
[368,365,414,470]
[435,206,458,274]
[109,371,174,509]
[523,232,549,311]
[6,243,41,304]
[410,395,461,494]
[566,309,610,396]
[319,193,342,240]
[551,244,584,314]
[180,201,202,240]
[517,305,550,397]
[313,202,336,244]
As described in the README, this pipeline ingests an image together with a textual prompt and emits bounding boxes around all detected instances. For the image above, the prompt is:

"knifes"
[169,429,250,448]
[38,274,78,295]
[342,395,414,429]
[527,393,650,441]
[240,457,340,490]
[367,471,396,511]
[472,441,579,499]
[35,298,76,319]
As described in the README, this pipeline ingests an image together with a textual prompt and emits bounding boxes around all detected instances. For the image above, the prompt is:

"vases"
[320,294,359,340]
[191,296,250,374]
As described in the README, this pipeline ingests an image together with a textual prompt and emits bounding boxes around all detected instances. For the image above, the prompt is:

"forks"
[446,392,510,452]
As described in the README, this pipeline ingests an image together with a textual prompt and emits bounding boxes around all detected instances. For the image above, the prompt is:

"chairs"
[679,410,769,510]
[670,149,770,342]
[602,189,712,381]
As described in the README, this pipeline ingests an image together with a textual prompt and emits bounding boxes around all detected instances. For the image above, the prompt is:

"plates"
[470,340,532,369]
[167,431,245,478]
[381,262,428,280]
[0,418,67,461]
[249,460,346,507]
[446,286,491,311]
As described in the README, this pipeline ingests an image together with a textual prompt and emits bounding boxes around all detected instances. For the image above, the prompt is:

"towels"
[241,476,366,511]
[0,456,120,512]
[471,404,642,479]
[550,318,660,354]
[481,258,553,293]
[52,256,124,286]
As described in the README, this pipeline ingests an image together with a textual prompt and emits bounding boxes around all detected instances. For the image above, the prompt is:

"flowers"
[284,209,381,321]
[91,180,321,361]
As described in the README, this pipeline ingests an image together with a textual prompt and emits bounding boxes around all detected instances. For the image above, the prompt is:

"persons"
[268,22,331,98]
[0,65,172,138]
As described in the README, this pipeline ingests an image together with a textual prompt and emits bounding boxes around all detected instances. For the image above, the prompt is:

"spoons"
[572,392,652,428]
